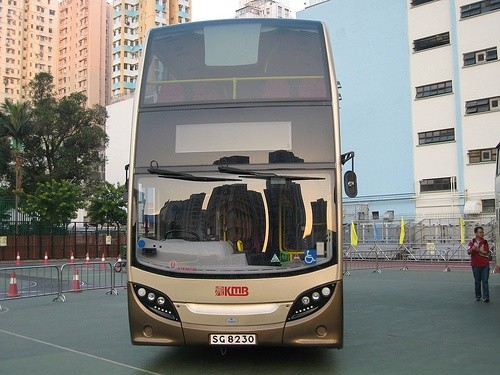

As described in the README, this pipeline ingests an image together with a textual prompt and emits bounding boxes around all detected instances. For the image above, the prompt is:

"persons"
[120,251,127,266]
[162,204,205,241]
[467,227,490,301]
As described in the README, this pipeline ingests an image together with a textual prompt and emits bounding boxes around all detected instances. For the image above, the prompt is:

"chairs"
[156,71,325,105]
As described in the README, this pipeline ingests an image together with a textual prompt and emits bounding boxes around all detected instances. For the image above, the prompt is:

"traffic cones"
[68,264,83,293]
[70,252,75,263]
[84,252,92,268]
[115,253,122,267]
[41,250,51,265]
[14,251,22,265]
[6,270,21,297]
[98,253,108,269]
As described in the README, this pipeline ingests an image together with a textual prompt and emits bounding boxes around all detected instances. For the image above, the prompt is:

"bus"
[492,142,500,270]
[122,15,361,353]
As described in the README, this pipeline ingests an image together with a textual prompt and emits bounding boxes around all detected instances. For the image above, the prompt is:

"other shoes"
[483,297,490,302]
[476,296,481,301]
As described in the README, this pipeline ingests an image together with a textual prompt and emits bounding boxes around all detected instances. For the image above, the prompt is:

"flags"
[350,221,358,246]
[459,213,465,243]
[398,216,405,244]
[373,221,379,241]
